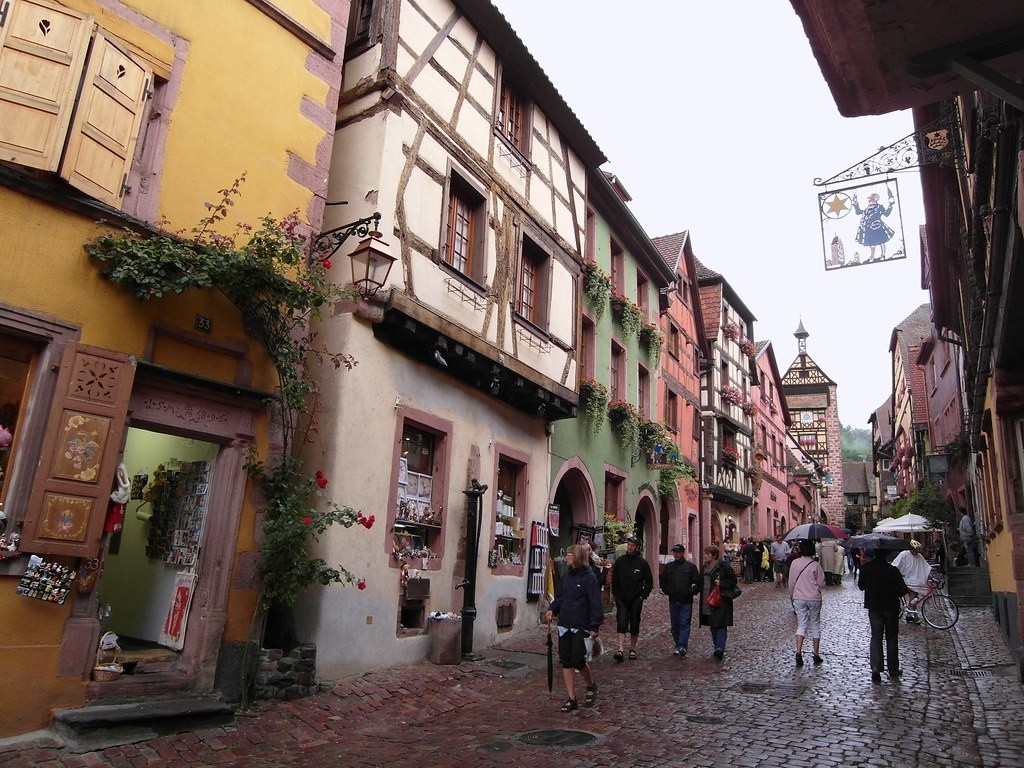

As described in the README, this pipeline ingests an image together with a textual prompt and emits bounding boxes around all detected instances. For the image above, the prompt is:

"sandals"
[561,695,578,712]
[629,649,637,660]
[585,681,598,704]
[614,651,624,662]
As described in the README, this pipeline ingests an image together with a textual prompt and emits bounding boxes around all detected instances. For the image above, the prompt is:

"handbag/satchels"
[717,566,742,601]
[704,585,724,609]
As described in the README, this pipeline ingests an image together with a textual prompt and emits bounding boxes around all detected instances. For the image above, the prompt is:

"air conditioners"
[883,459,890,472]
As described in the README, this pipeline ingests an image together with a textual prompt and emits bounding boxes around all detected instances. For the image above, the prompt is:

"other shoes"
[673,649,679,655]
[775,582,781,588]
[813,654,823,663]
[679,648,687,659]
[906,613,922,624]
[889,666,903,676]
[871,666,882,682]
[796,653,803,665]
[714,650,723,661]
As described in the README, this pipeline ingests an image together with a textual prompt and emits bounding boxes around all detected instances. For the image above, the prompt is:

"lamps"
[307,212,400,304]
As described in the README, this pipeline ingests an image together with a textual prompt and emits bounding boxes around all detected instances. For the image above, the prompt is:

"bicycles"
[898,576,959,630]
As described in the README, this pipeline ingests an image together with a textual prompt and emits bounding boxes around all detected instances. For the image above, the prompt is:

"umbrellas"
[780,511,945,547]
[547,617,554,699]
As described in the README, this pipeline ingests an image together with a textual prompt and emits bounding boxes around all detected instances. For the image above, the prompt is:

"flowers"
[723,321,740,342]
[741,402,758,418]
[749,463,763,496]
[602,506,633,532]
[739,336,756,359]
[789,417,826,469]
[584,256,666,371]
[721,384,745,406]
[890,445,923,497]
[721,446,740,469]
[578,374,666,454]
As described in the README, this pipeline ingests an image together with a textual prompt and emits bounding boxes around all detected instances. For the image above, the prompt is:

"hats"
[671,544,685,552]
[949,542,958,553]
[627,537,640,547]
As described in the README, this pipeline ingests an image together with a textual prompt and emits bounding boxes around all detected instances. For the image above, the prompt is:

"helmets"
[910,539,923,552]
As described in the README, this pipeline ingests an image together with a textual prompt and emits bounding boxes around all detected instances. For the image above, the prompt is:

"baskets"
[92,631,124,682]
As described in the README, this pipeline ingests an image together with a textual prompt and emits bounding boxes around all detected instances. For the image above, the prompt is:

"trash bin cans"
[428,613,461,665]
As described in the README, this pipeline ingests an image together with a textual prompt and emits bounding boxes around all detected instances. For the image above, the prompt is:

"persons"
[699,546,736,657]
[857,548,909,680]
[935,507,980,576]
[723,531,869,589]
[787,540,826,665]
[890,539,932,623]
[659,544,702,656]
[546,541,610,713]
[610,537,653,660]
